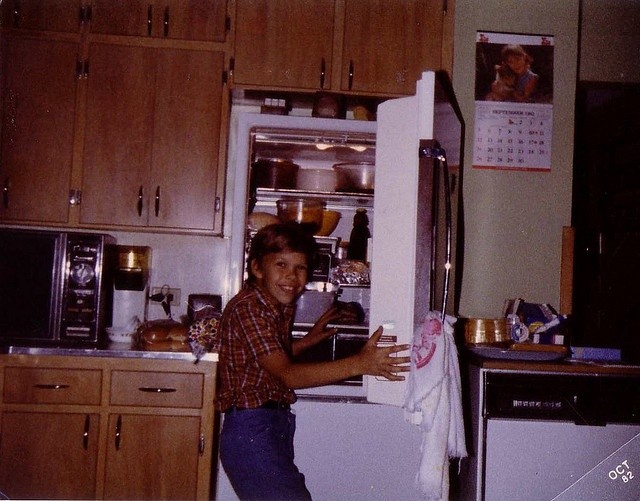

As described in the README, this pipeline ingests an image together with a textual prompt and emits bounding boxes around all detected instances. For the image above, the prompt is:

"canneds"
[116,241,150,290]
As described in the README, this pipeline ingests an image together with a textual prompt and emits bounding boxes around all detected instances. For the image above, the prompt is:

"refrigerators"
[231,78,449,497]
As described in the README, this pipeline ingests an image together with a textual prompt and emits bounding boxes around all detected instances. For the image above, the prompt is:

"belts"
[223,399,292,412]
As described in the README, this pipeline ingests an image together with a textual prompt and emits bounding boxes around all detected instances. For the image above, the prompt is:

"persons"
[214,223,414,501]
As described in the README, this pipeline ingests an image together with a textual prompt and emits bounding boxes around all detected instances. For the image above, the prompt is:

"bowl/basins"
[274,196,323,233]
[332,158,374,193]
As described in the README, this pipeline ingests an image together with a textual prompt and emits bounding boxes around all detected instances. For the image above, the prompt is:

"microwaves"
[0,223,114,351]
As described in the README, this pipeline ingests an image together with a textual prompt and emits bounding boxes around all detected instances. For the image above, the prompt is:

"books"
[501,297,524,319]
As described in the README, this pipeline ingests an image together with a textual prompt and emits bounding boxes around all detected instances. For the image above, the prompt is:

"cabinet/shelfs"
[1,359,220,501]
[230,1,454,98]
[1,0,227,42]
[85,40,228,236]
[4,33,86,229]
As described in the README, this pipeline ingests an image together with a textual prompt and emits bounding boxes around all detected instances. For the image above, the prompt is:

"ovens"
[466,367,639,496]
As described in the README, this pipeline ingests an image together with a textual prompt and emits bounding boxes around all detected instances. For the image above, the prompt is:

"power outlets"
[152,287,180,307]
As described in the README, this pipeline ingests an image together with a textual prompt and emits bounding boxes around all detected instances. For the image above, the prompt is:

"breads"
[137,319,189,352]
[248,211,277,232]
